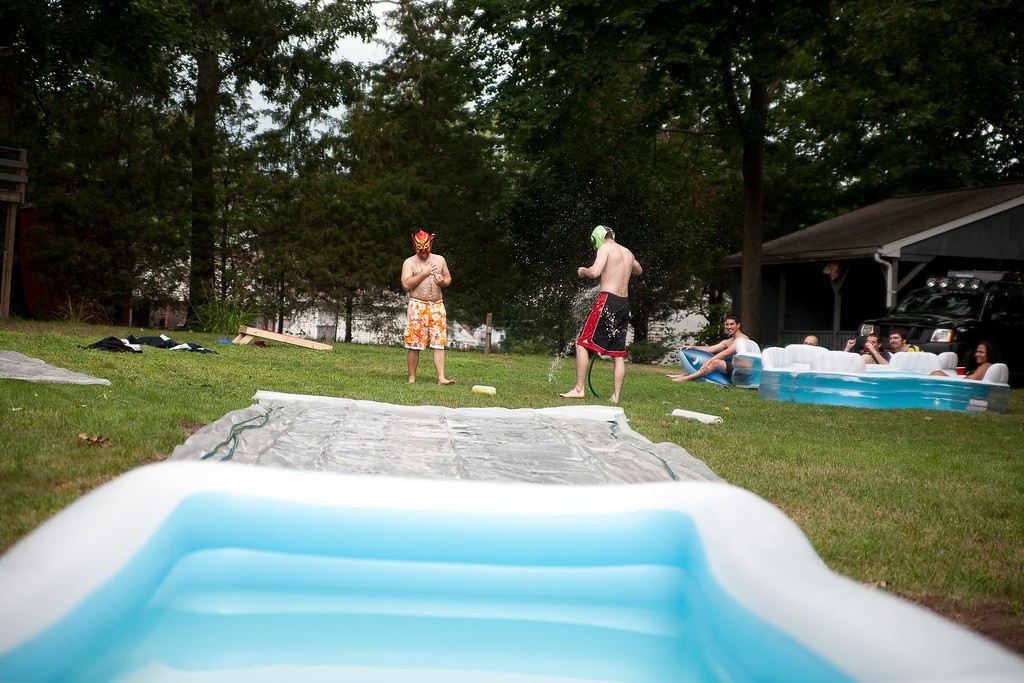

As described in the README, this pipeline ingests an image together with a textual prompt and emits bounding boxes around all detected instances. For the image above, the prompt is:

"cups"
[864,345,869,352]
[956,367,966,375]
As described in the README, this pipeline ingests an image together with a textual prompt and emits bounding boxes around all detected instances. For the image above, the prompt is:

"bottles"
[472,385,496,395]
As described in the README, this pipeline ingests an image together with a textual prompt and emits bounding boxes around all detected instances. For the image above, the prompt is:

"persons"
[666,315,754,386]
[889,330,924,352]
[844,333,892,364]
[560,225,642,403]
[401,231,455,385]
[803,335,818,346]
[929,341,994,381]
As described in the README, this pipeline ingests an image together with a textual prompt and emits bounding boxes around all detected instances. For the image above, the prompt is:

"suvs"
[849,270,1024,388]
